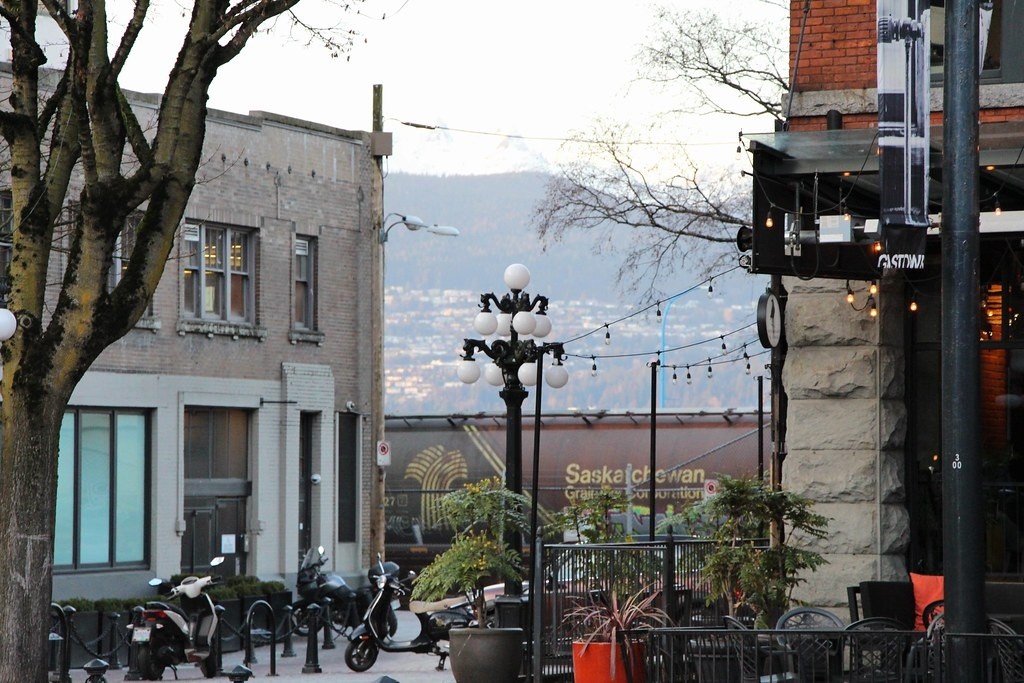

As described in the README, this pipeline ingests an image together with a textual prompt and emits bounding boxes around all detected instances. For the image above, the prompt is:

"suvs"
[482,535,741,651]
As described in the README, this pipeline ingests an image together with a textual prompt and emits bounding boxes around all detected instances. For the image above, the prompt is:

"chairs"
[848,571,944,683]
[719,598,1024,683]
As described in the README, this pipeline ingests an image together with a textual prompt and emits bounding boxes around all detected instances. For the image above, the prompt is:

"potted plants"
[410,475,677,683]
[46,575,292,670]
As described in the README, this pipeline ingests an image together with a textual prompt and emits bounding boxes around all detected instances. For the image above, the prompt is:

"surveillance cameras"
[347,400,355,411]
[311,474,321,485]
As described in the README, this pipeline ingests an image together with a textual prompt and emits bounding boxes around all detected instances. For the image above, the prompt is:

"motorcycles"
[290,545,399,638]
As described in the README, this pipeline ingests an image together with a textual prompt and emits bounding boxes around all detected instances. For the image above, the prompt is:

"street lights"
[457,263,569,676]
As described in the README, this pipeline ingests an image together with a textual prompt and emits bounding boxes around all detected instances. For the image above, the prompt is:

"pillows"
[906,572,945,630]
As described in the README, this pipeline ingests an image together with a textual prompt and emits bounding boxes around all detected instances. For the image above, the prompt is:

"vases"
[691,652,759,683]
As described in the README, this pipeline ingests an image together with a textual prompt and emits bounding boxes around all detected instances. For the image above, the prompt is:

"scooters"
[344,552,479,671]
[131,556,225,681]
